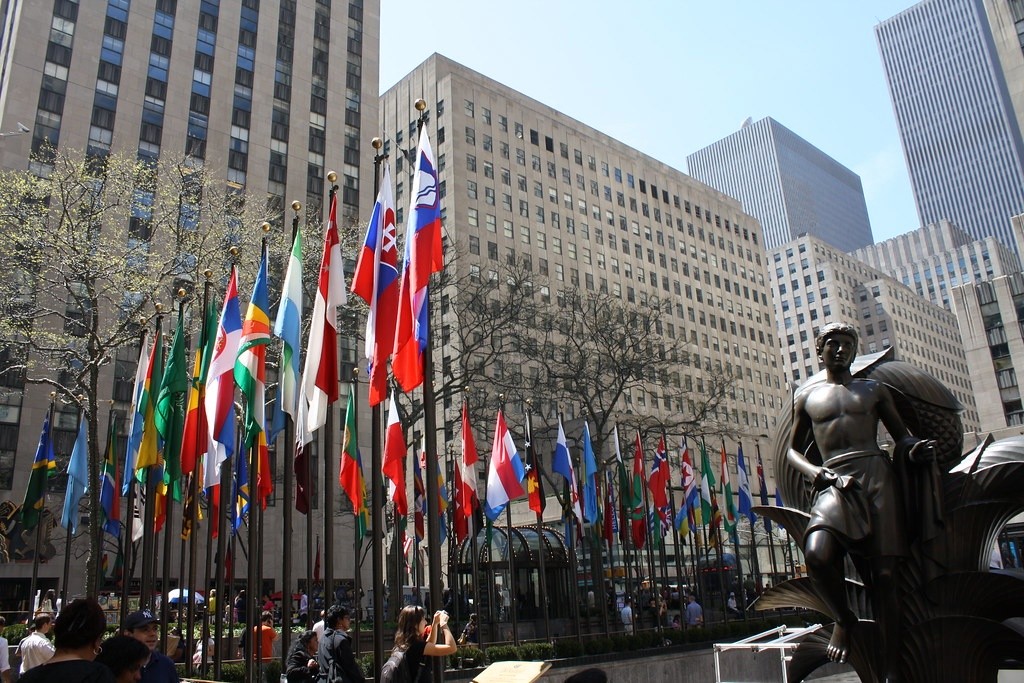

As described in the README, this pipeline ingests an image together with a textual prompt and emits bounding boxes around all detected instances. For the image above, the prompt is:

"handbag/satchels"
[327,630,365,683]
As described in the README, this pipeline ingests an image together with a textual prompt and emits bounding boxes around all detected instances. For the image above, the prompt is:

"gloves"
[439,610,449,627]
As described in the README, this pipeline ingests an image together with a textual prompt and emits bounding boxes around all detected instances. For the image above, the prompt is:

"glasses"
[131,625,159,632]
[125,663,145,674]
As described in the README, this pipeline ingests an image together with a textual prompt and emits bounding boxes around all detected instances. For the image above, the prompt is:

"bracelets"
[440,623,448,628]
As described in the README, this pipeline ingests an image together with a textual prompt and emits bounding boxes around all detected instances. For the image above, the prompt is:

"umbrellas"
[167,588,204,605]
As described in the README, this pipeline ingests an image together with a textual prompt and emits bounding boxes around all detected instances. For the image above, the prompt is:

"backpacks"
[380,639,426,683]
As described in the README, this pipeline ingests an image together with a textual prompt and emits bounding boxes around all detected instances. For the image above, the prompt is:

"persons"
[34,586,67,619]
[195,625,215,663]
[0,616,12,683]
[238,611,278,663]
[787,324,937,683]
[208,587,311,628]
[170,627,186,663]
[14,613,56,680]
[410,587,422,607]
[727,575,772,615]
[380,603,457,683]
[286,605,364,683]
[603,583,703,636]
[21,598,105,683]
[440,583,501,622]
[94,609,178,683]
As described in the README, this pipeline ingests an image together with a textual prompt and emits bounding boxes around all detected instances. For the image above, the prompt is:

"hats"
[124,609,161,628]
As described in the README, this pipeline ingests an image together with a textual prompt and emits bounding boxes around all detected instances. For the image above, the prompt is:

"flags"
[19,119,441,542]
[439,398,786,552]
